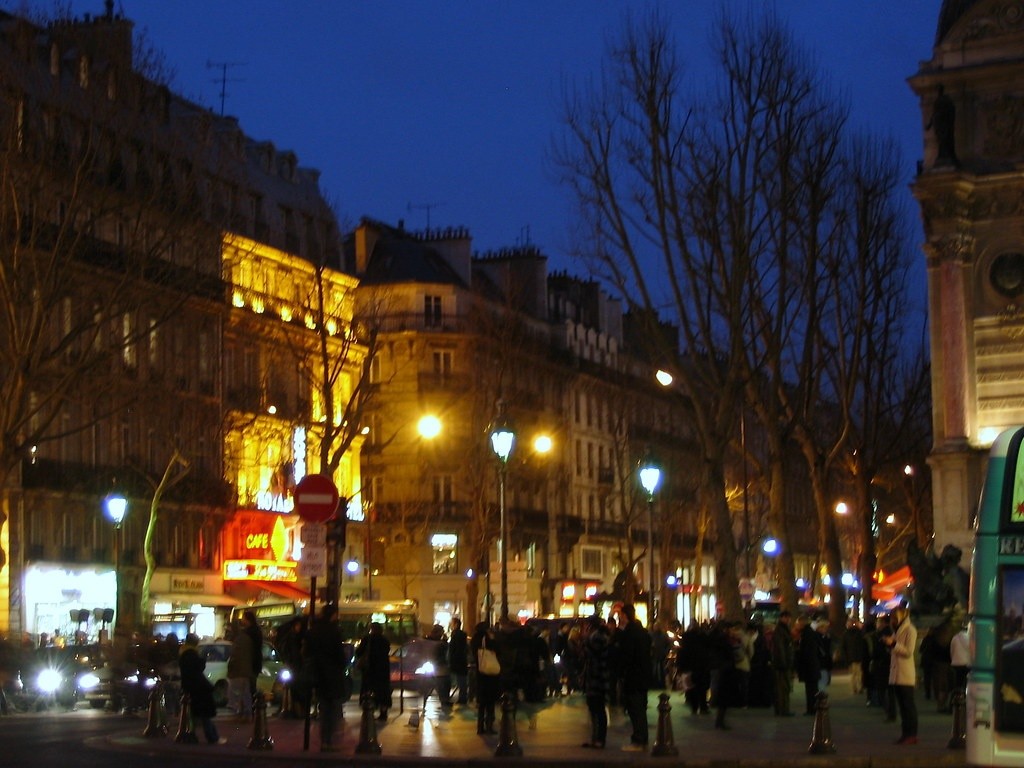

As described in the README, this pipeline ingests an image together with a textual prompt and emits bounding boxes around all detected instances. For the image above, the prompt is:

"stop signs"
[293,474,340,526]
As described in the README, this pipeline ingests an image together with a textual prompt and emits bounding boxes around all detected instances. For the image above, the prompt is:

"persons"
[948,620,969,695]
[0,606,946,747]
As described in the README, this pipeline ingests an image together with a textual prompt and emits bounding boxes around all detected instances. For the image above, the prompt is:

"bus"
[230,600,419,657]
[963,425,1024,768]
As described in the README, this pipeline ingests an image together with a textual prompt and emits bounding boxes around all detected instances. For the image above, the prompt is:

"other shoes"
[476,727,485,734]
[378,714,387,720]
[631,735,648,745]
[897,734,917,745]
[486,727,498,734]
[775,709,795,717]
[700,710,711,715]
[715,721,730,730]
[212,736,227,744]
[320,742,339,753]
[582,741,604,749]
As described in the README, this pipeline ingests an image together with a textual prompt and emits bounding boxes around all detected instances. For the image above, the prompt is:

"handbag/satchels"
[477,636,500,674]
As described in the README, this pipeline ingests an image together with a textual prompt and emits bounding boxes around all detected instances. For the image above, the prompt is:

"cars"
[387,637,449,695]
[15,639,286,717]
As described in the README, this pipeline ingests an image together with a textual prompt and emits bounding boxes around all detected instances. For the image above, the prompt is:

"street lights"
[636,446,660,640]
[103,478,128,648]
[489,398,514,623]
